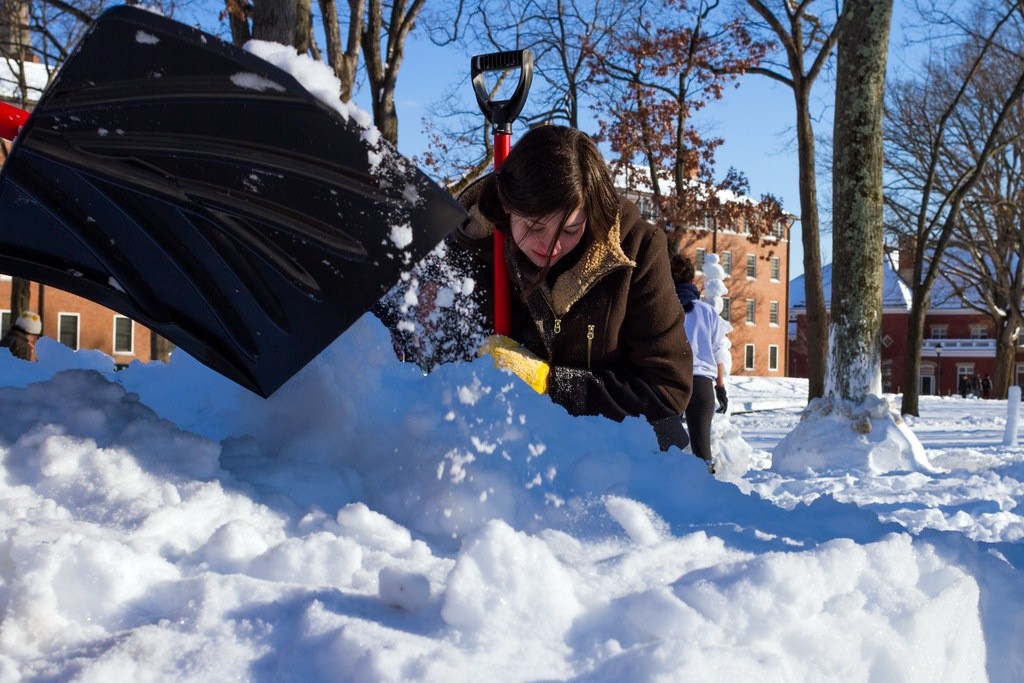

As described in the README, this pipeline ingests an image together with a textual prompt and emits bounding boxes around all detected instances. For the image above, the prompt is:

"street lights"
[934,342,943,397]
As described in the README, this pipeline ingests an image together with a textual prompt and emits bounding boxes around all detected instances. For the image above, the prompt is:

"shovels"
[1,5,471,402]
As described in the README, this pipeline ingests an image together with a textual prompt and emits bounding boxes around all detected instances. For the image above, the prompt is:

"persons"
[429,124,693,451]
[961,372,992,399]
[0,311,42,361]
[671,255,728,472]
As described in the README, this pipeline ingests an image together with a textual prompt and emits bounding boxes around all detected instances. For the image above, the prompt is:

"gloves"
[478,334,549,395]
[715,385,727,414]
